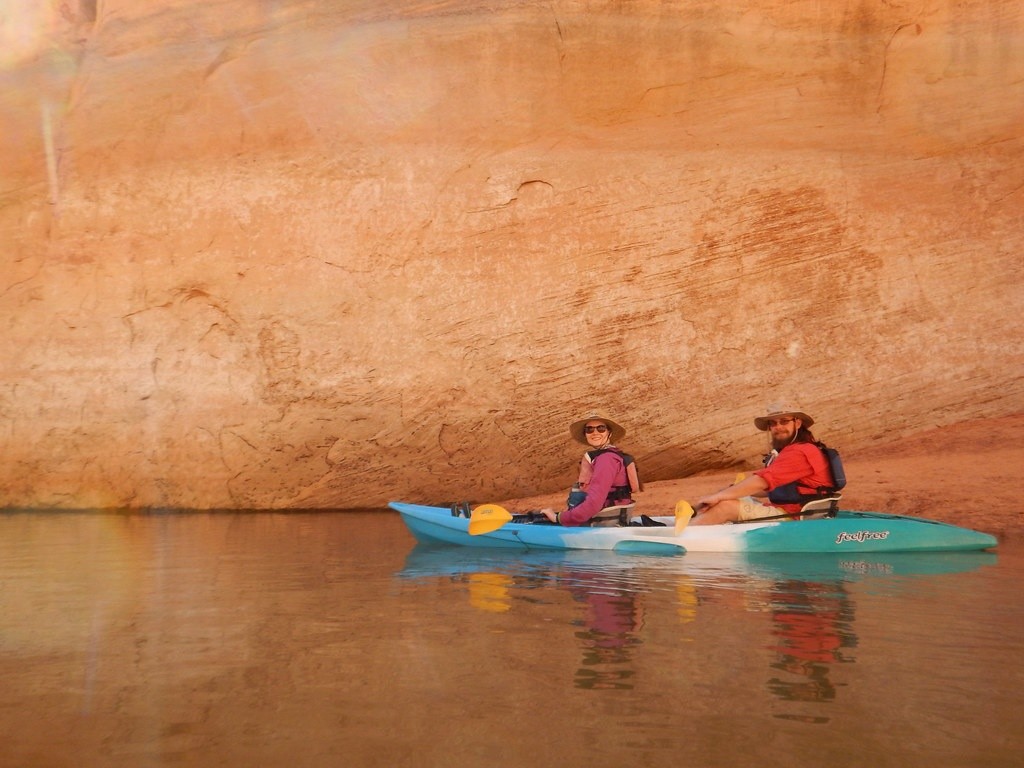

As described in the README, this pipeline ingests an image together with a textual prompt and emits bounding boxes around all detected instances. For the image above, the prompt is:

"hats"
[569,411,626,444]
[754,402,814,431]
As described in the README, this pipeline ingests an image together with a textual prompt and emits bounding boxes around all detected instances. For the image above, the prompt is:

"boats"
[388,501,997,552]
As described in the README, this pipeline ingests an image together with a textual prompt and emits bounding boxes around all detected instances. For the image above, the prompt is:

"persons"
[451,410,640,527]
[631,400,847,526]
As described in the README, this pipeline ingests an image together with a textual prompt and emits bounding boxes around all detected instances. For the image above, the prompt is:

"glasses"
[768,418,795,426]
[584,425,608,434]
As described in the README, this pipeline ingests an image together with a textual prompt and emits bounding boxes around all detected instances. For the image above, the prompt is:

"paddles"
[674,471,746,536]
[468,503,560,537]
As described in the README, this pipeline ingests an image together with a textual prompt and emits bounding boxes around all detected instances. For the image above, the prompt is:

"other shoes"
[451,504,460,518]
[641,514,667,526]
[462,502,472,517]
